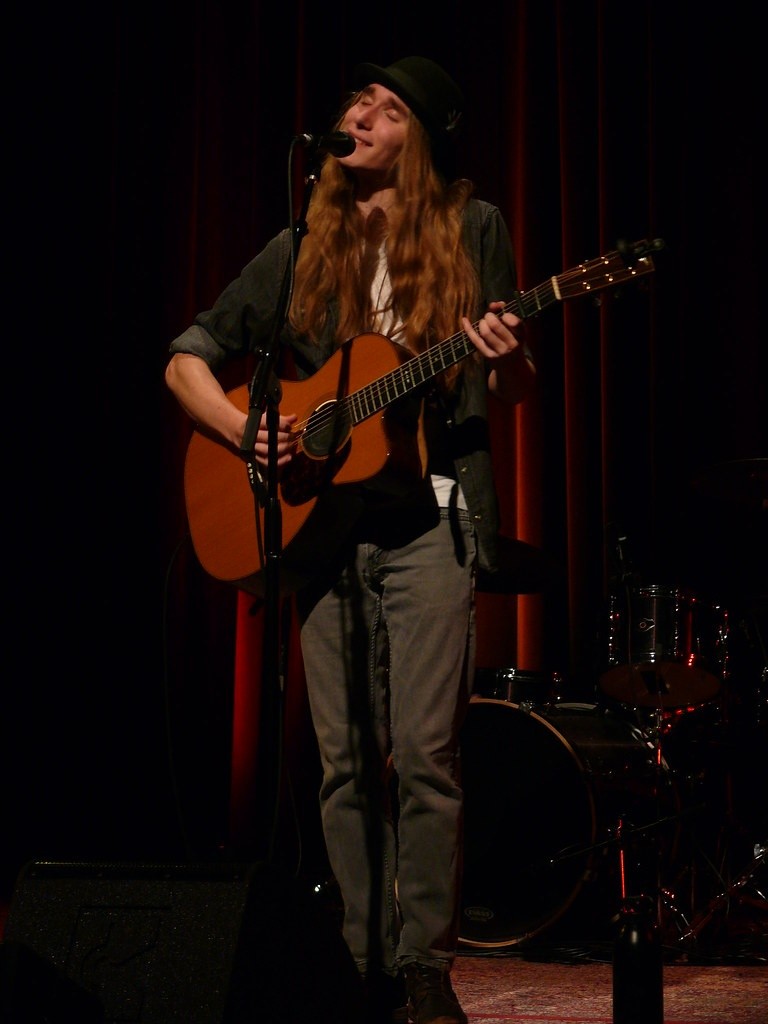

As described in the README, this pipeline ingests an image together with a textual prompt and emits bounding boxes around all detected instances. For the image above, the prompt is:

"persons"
[164,58,542,1024]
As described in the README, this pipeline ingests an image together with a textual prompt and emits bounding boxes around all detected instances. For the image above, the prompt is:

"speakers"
[0,859,371,1024]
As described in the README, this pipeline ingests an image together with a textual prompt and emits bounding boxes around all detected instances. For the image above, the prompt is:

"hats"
[361,56,468,189]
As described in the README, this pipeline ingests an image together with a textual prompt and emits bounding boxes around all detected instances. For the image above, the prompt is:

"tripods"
[636,669,768,962]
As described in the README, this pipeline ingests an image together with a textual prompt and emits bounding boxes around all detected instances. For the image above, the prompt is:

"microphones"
[298,131,357,158]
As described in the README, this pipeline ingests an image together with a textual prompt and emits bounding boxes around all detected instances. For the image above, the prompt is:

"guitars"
[179,231,682,585]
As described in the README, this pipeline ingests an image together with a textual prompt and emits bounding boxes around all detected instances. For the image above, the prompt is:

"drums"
[594,585,733,718]
[456,674,603,952]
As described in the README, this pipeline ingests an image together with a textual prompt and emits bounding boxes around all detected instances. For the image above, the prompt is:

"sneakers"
[406,960,468,1024]
[389,999,408,1020]
